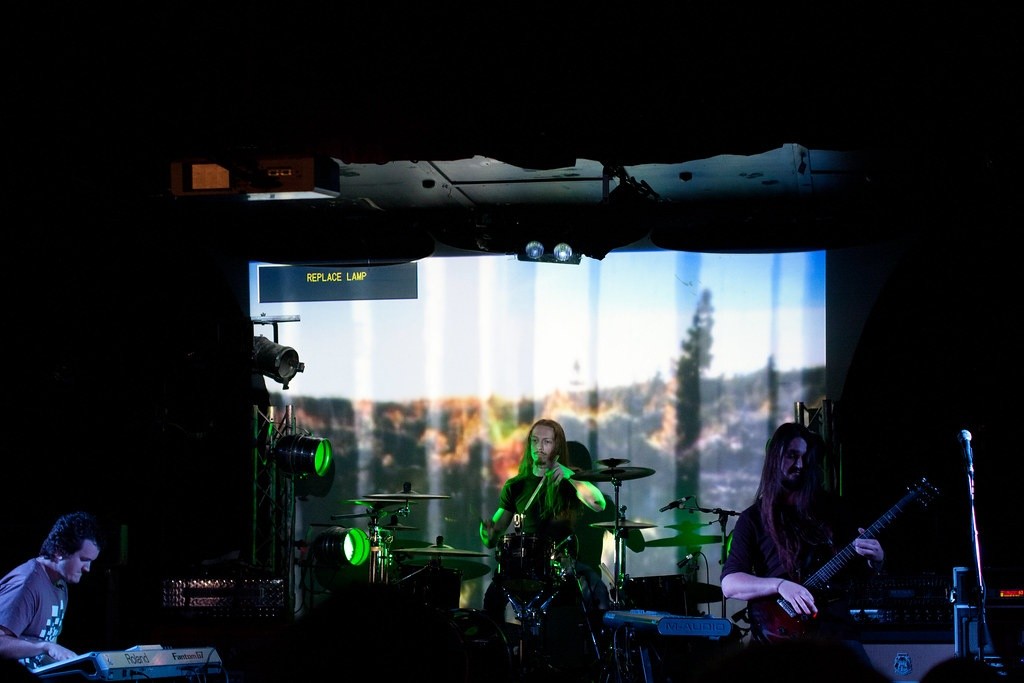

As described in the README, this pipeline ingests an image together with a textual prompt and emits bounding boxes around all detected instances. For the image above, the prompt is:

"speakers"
[859,630,953,683]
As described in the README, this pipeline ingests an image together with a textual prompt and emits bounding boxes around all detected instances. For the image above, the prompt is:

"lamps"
[313,525,372,569]
[273,433,334,480]
[517,229,579,265]
[255,335,304,393]
[246,155,345,202]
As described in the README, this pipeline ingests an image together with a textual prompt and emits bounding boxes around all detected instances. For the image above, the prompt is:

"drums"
[496,534,560,589]
[442,607,512,671]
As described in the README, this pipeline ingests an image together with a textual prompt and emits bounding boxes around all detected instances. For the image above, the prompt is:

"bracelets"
[777,579,785,592]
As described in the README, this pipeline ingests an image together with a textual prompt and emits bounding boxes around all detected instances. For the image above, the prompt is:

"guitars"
[746,470,942,645]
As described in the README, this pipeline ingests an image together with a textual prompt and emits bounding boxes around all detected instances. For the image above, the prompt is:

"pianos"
[30,642,224,683]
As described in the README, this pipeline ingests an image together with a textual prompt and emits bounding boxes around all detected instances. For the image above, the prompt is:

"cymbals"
[570,458,654,482]
[348,498,418,509]
[393,542,489,557]
[362,489,452,499]
[589,520,656,533]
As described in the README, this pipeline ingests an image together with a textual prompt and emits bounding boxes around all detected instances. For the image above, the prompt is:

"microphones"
[676,552,698,568]
[659,495,692,512]
[555,535,576,551]
[956,429,975,478]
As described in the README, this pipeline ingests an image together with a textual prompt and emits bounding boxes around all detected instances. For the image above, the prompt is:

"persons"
[719,423,887,683]
[0,513,101,683]
[479,421,606,624]
[559,442,645,610]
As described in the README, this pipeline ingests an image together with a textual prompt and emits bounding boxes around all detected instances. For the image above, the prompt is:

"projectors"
[170,153,340,199]
